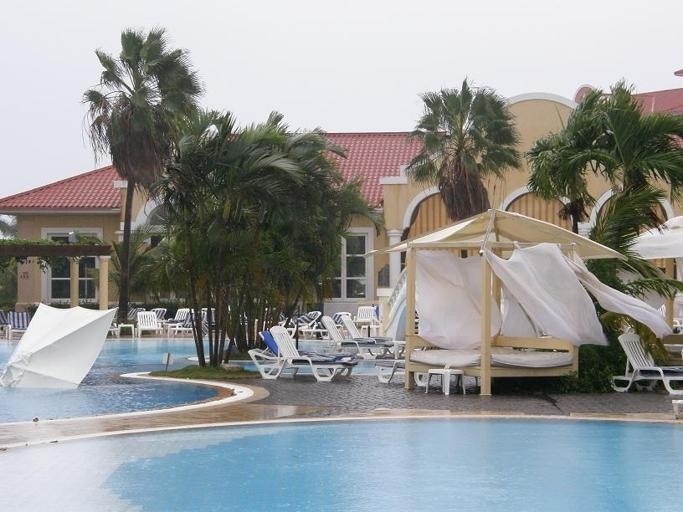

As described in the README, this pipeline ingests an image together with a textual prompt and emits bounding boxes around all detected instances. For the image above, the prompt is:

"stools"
[424,369,466,396]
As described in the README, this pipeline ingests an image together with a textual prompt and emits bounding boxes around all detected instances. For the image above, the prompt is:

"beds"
[403,242,579,397]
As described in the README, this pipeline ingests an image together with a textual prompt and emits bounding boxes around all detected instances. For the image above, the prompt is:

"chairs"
[246,306,438,387]
[0,310,30,341]
[607,333,682,396]
[108,307,221,339]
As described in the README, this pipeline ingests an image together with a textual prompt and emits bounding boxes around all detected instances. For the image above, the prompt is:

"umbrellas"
[620,216,682,260]
[0,301,118,393]
